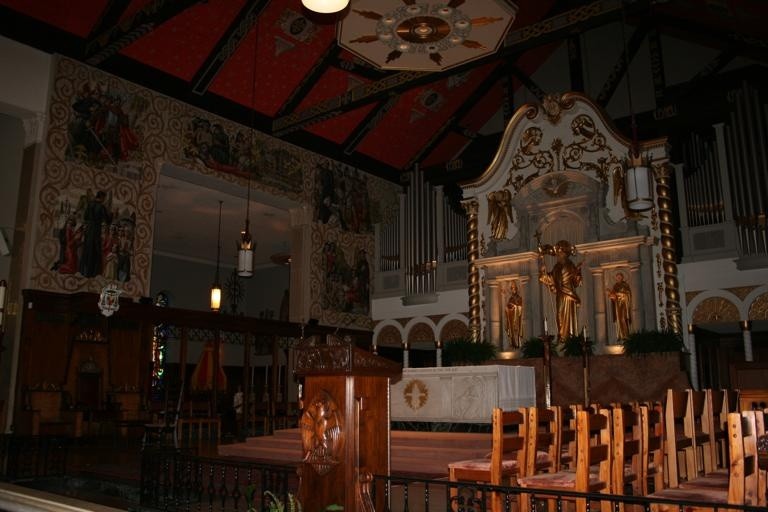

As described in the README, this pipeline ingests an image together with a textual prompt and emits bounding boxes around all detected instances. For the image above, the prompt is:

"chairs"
[149,396,221,442]
[19,381,151,443]
[248,398,299,436]
[447,378,768,512]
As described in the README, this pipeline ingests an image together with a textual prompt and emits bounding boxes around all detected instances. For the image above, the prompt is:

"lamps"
[207,115,258,313]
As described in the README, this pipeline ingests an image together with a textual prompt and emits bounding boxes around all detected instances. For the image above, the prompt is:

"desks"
[390,364,534,432]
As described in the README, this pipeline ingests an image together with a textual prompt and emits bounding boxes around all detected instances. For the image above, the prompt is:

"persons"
[538,239,583,341]
[608,272,632,340]
[505,281,524,349]
